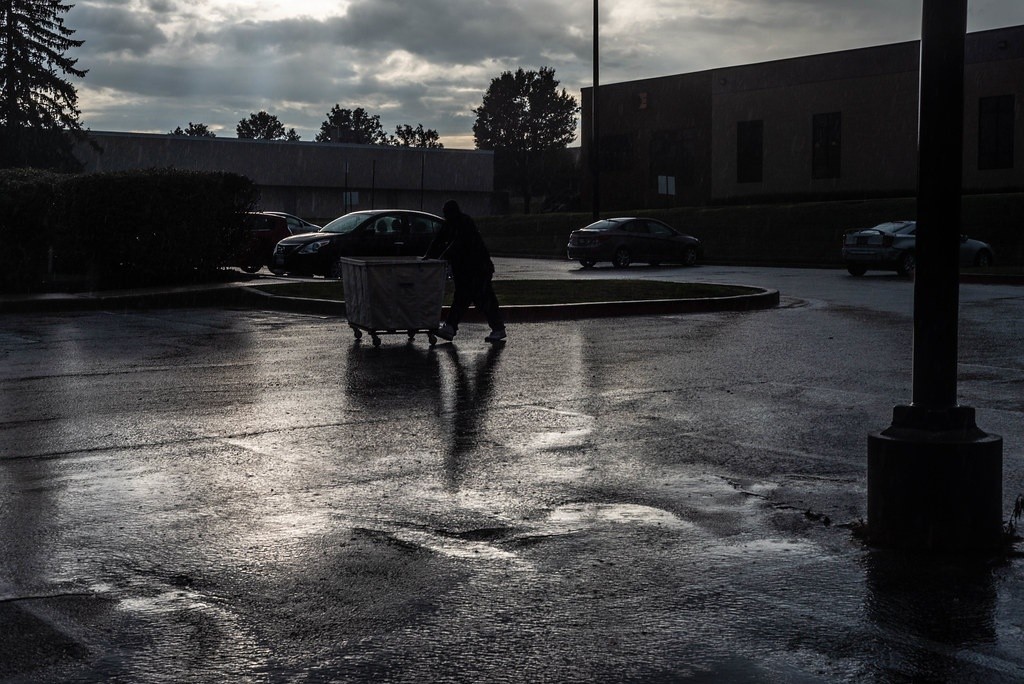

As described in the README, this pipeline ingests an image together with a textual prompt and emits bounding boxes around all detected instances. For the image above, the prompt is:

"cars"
[841,219,996,281]
[270,208,447,280]
[245,209,321,258]
[567,216,704,271]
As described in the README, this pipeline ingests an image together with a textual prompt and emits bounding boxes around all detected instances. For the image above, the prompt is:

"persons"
[421,199,507,341]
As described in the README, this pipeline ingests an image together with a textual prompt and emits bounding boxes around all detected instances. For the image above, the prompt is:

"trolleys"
[342,254,448,348]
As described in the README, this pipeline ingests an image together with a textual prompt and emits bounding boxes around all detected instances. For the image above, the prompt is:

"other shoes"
[433,325,456,341]
[485,326,507,343]
